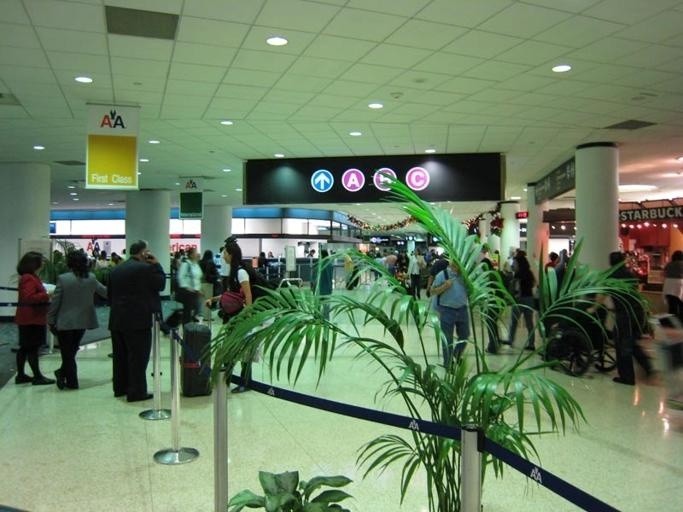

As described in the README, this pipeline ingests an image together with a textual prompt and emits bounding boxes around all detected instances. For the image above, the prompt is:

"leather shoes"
[55,369,66,390]
[613,376,635,385]
[15,375,32,384]
[32,376,55,385]
[128,394,153,402]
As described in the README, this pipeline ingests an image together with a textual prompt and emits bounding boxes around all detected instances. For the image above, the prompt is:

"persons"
[344,247,437,301]
[425,250,471,373]
[662,250,683,322]
[307,248,316,257]
[15,252,53,383]
[480,247,653,385]
[46,235,275,401]
[298,250,309,258]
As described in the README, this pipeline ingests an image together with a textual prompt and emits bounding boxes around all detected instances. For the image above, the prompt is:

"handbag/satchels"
[221,292,245,313]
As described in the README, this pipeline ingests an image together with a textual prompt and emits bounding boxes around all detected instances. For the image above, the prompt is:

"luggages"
[181,304,214,398]
